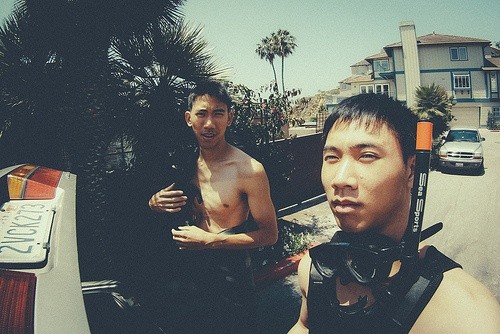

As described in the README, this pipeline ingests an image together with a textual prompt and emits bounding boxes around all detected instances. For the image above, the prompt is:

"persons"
[143,80,278,334]
[284,94,499,334]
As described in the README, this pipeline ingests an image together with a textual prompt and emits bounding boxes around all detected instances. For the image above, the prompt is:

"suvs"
[438,128,485,173]
